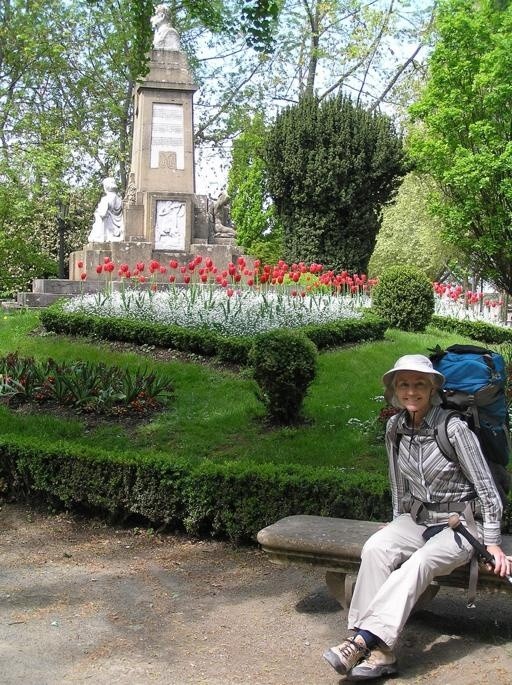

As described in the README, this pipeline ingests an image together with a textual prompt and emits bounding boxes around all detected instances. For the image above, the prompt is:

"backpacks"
[391,344,509,495]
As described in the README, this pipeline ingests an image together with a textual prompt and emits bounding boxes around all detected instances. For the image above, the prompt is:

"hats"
[382,353,445,387]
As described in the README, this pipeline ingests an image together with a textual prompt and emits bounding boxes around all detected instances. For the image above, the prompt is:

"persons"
[322,354,511,681]
[87,177,124,243]
[146,5,181,52]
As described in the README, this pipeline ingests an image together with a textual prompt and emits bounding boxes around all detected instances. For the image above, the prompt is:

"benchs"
[257,515,512,617]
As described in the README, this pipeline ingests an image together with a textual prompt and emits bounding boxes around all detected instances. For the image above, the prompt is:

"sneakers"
[324,635,367,674]
[348,649,400,682]
